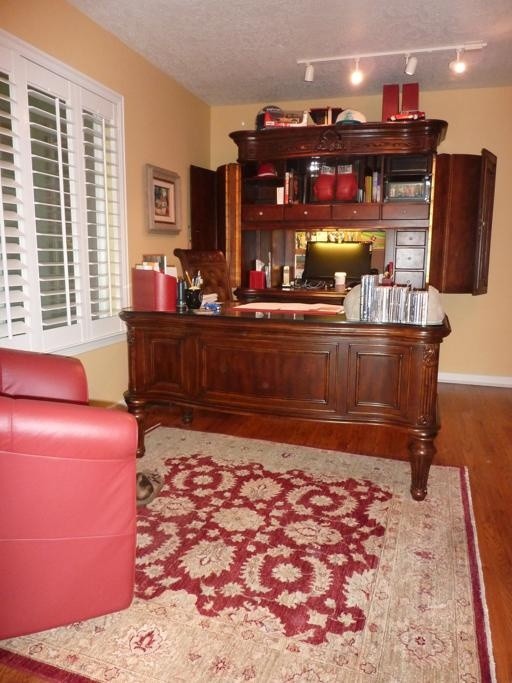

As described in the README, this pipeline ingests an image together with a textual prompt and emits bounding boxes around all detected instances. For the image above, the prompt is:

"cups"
[334,271,347,291]
[185,288,202,308]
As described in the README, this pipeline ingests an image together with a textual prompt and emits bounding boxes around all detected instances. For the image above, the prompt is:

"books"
[360,271,428,325]
[284,169,300,204]
[358,171,381,203]
[134,252,167,274]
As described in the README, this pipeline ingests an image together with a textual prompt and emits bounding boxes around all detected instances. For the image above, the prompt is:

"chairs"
[0,346,139,642]
[172,246,237,304]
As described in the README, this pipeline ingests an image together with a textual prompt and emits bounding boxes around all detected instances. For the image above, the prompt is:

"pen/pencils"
[185,267,203,289]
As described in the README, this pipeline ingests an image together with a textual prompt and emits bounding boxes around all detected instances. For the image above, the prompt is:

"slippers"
[136,471,163,506]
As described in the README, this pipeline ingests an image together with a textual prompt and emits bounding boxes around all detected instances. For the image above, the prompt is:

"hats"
[335,109,367,124]
[246,161,281,183]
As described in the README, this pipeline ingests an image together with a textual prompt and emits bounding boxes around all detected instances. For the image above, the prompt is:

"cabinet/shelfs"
[120,117,498,501]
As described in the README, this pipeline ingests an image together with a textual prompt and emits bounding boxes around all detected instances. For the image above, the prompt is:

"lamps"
[297,41,486,85]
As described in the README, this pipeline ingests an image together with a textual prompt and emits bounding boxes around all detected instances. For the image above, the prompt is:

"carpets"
[0,421,499,683]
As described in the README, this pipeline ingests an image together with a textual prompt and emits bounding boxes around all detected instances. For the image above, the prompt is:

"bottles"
[177,279,186,307]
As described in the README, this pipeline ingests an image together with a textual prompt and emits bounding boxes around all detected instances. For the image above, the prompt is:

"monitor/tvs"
[305,241,369,291]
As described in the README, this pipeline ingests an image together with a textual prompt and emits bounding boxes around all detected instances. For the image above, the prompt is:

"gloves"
[312,165,358,202]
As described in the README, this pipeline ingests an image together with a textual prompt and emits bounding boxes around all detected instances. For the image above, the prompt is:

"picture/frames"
[145,165,181,233]
